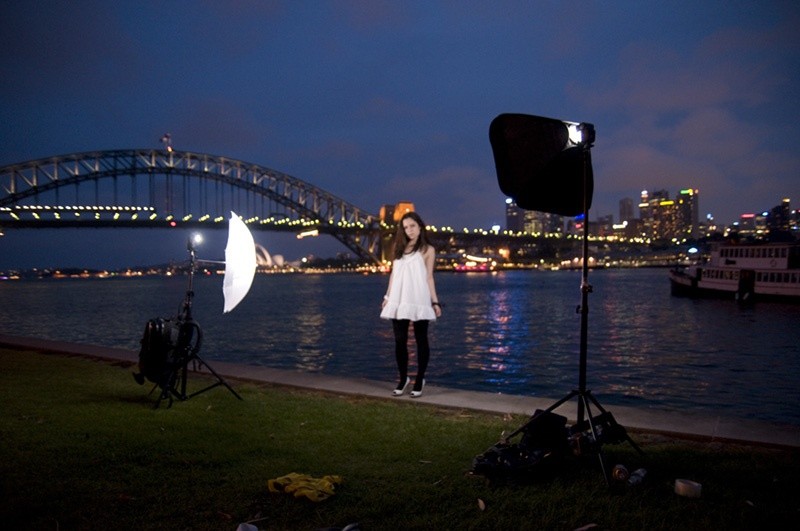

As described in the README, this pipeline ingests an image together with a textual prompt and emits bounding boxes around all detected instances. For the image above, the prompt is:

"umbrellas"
[196,209,259,316]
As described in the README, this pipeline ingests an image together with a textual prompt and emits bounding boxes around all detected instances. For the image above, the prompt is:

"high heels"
[391,376,411,396]
[409,378,426,397]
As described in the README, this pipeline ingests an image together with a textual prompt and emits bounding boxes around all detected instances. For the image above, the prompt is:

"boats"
[666,241,800,300]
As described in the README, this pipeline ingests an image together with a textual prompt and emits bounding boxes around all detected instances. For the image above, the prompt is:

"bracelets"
[432,302,440,306]
[383,295,389,299]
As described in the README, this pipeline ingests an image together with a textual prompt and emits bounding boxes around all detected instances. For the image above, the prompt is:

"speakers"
[139,319,180,381]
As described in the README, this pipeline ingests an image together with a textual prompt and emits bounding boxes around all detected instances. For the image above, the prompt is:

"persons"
[379,212,443,397]
